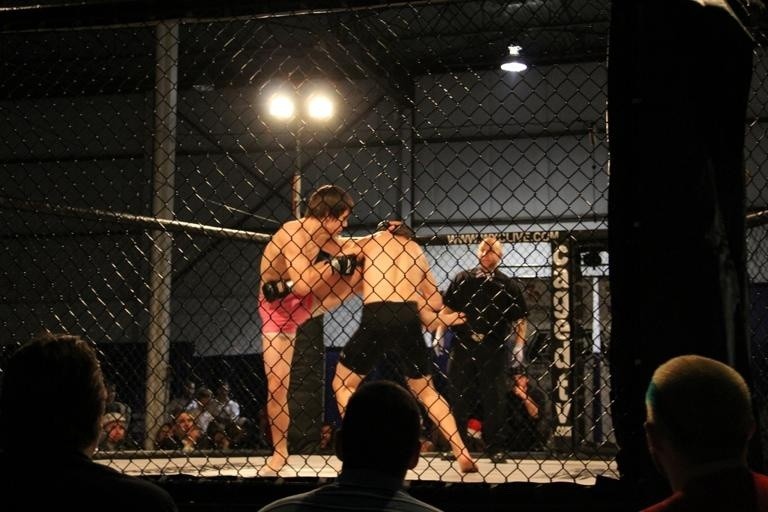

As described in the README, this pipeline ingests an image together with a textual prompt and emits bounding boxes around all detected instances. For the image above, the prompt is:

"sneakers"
[440,450,455,460]
[491,451,505,463]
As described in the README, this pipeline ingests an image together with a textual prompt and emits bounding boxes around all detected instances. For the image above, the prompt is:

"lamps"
[497,34,529,73]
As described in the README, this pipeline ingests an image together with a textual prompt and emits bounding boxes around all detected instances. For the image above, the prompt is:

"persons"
[498,370,544,451]
[434,236,527,465]
[257,380,446,511]
[264,220,478,471]
[314,425,337,451]
[1,333,176,510]
[257,185,464,473]
[634,354,767,510]
[99,381,259,448]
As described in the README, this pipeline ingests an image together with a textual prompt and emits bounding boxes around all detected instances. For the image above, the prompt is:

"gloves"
[262,278,294,303]
[331,256,356,276]
